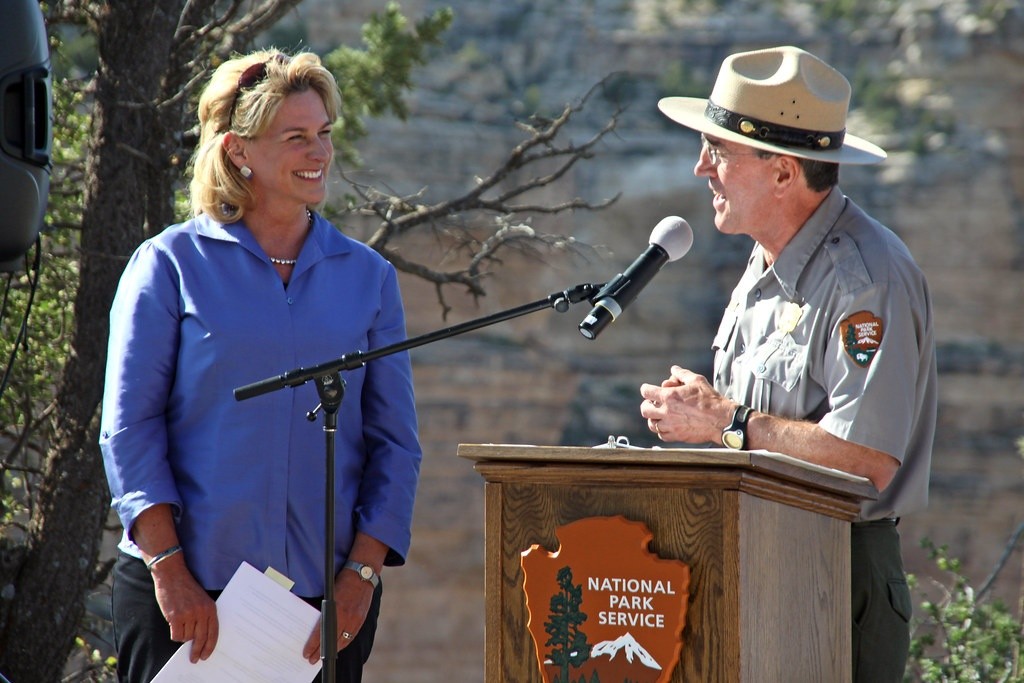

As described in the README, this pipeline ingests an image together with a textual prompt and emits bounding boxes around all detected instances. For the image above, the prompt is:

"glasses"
[696,134,772,165]
[226,54,291,132]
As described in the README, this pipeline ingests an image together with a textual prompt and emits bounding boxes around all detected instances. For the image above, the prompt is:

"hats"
[658,47,890,167]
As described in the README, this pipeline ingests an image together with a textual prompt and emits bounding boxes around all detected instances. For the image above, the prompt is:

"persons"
[97,51,423,683]
[641,45,938,683]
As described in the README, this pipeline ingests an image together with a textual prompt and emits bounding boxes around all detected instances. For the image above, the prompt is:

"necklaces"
[267,210,313,265]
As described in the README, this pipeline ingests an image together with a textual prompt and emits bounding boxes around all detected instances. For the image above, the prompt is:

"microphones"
[579,216,694,340]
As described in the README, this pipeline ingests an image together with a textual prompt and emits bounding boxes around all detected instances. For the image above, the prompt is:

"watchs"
[722,405,755,451]
[344,561,379,588]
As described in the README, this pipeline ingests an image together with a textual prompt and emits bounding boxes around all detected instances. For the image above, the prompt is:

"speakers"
[0,0,52,272]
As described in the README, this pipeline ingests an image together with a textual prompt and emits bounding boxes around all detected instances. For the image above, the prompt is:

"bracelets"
[147,546,182,570]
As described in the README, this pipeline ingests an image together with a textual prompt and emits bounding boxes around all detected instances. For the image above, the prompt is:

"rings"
[342,632,352,639]
[655,423,661,433]
